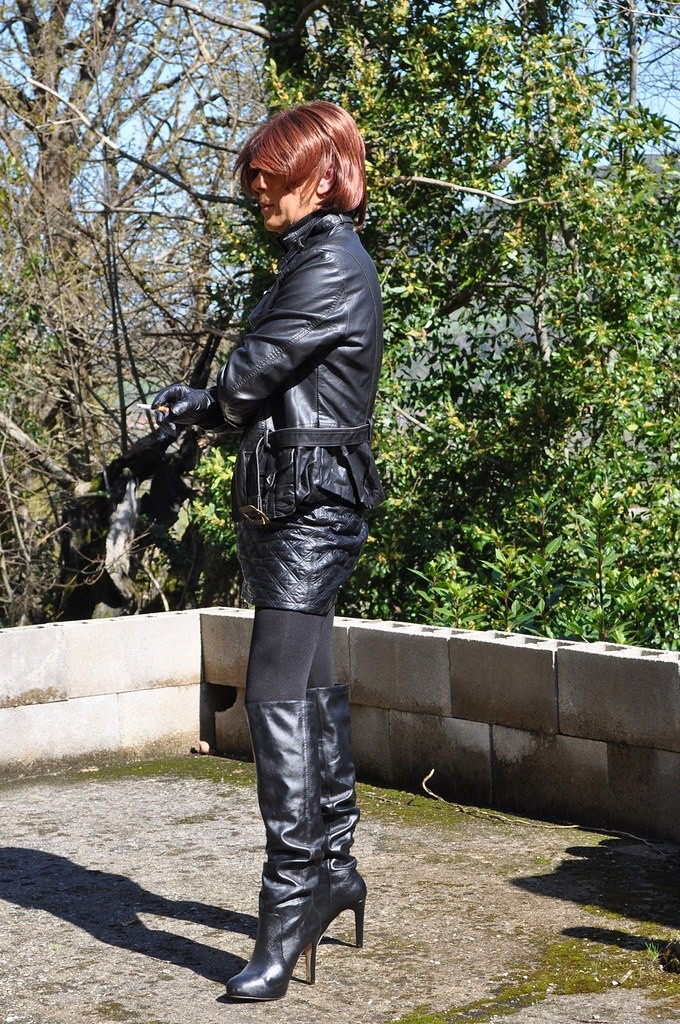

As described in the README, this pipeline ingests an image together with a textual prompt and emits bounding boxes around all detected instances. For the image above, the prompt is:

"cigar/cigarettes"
[137,403,169,413]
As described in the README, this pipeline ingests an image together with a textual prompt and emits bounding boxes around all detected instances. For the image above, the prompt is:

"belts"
[238,422,369,526]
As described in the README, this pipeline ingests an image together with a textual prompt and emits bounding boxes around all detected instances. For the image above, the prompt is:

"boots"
[305,682,367,948]
[225,699,323,1001]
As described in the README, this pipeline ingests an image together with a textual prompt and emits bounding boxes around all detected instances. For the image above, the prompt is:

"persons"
[151,101,387,1001]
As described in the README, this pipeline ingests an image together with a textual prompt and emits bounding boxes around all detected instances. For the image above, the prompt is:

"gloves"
[150,384,230,440]
